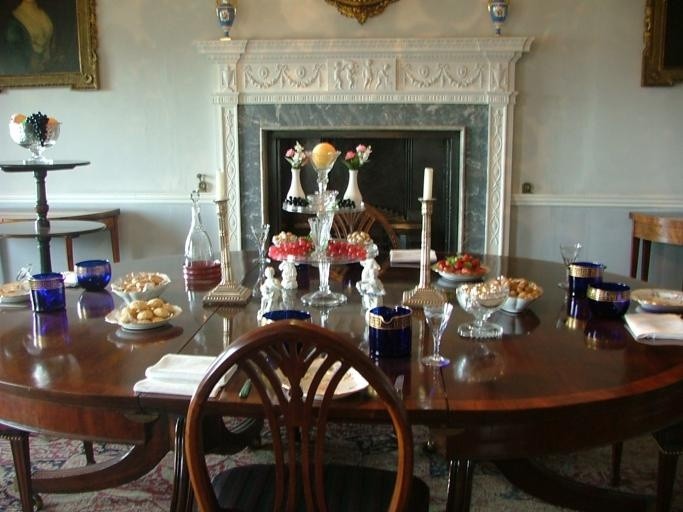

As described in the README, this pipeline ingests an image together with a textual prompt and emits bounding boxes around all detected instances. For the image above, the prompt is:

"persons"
[4,0,61,74]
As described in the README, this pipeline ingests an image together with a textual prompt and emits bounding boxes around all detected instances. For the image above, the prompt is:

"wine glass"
[304,150,342,192]
[422,303,453,367]
[253,264,266,298]
[557,243,582,288]
[250,225,270,263]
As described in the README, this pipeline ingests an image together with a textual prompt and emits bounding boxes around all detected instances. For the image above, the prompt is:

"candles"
[422,167,434,197]
[216,171,228,199]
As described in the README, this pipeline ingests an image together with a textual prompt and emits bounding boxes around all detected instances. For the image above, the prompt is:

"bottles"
[184,190,214,270]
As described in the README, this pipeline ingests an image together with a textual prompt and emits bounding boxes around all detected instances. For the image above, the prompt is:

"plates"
[104,270,182,329]
[0,293,30,302]
[631,288,683,312]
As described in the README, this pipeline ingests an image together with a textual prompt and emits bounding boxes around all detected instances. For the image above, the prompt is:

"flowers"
[342,145,371,168]
[286,141,311,168]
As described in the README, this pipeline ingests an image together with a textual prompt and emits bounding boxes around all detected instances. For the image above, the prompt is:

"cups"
[565,296,590,331]
[29,272,65,312]
[369,306,413,358]
[33,313,70,349]
[566,262,606,298]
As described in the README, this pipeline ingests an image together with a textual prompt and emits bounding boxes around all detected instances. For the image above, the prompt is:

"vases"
[341,170,362,207]
[285,168,305,203]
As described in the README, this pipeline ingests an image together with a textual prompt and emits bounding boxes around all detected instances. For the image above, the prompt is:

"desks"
[5,209,123,271]
[629,212,683,284]
[1,158,109,283]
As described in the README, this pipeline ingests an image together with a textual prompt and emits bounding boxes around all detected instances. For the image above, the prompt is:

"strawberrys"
[437,253,487,274]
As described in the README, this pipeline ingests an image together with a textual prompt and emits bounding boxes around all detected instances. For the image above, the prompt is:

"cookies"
[120,297,175,323]
[124,275,163,291]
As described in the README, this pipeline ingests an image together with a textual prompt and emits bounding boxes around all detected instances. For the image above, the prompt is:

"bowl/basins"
[258,309,311,325]
[436,273,539,314]
[75,259,112,290]
[583,317,627,351]
[586,282,630,317]
[76,290,115,319]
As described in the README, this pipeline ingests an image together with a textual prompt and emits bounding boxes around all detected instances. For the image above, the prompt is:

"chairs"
[322,201,400,282]
[168,320,430,510]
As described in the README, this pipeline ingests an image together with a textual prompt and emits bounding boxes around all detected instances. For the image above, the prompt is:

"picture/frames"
[642,1,682,86]
[0,0,101,91]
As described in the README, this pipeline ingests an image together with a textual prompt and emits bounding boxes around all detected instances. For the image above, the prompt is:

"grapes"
[285,196,308,206]
[26,111,48,146]
[337,199,355,209]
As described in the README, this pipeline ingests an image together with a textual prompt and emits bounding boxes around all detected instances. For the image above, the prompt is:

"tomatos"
[327,240,366,261]
[277,239,314,255]
